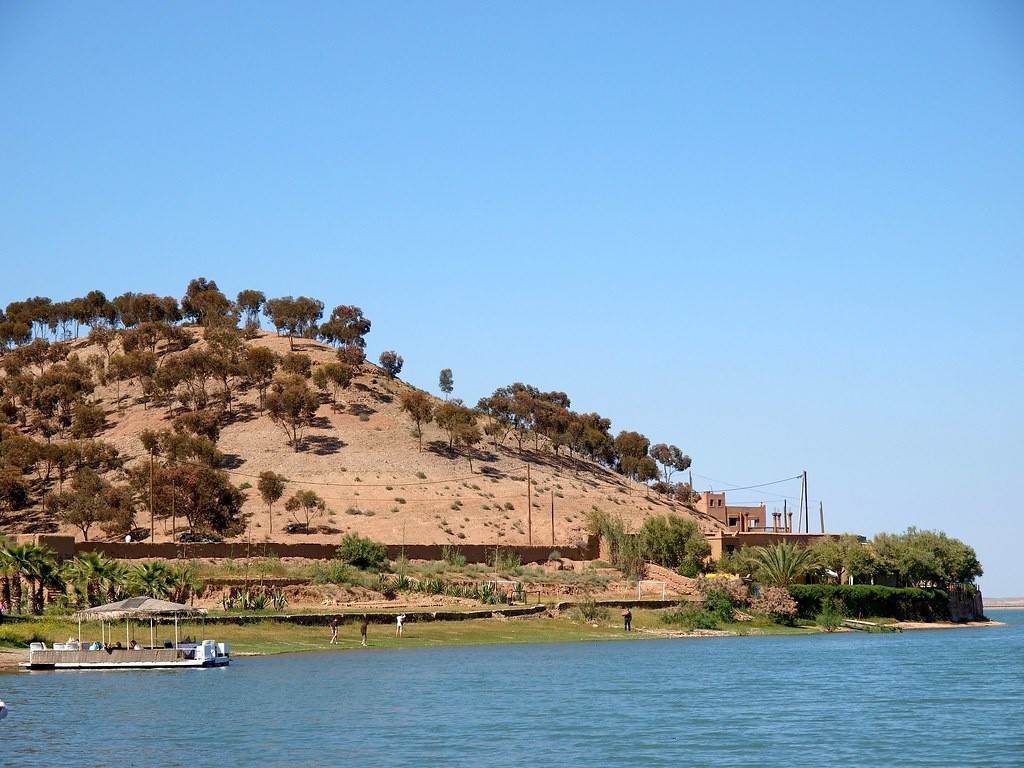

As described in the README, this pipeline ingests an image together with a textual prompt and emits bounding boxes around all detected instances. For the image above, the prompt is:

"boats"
[16,593,237,671]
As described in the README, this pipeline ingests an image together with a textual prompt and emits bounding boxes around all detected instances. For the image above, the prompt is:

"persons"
[360,620,370,647]
[396,613,406,637]
[330,617,340,645]
[124,534,135,542]
[622,608,632,631]
[130,640,143,650]
[507,588,514,606]
[109,642,124,650]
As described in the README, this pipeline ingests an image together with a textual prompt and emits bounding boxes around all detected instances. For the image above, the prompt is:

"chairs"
[163,640,173,649]
[30,642,49,659]
[54,643,89,651]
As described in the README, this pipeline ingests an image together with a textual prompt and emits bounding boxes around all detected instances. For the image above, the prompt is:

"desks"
[174,643,198,658]
